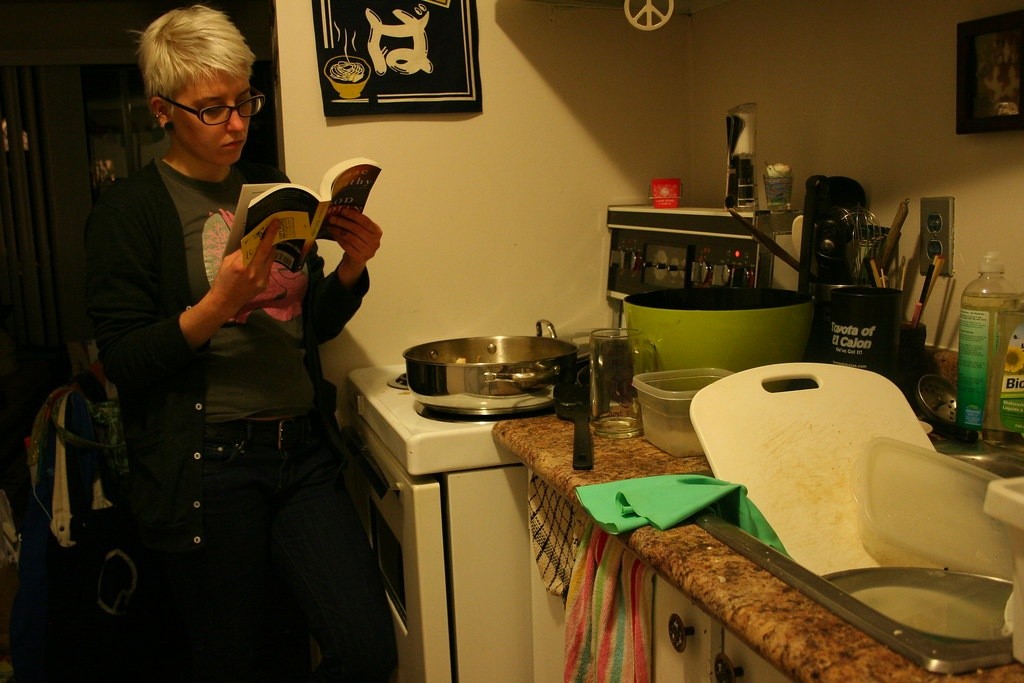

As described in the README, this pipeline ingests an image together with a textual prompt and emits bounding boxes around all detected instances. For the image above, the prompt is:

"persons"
[82,5,397,683]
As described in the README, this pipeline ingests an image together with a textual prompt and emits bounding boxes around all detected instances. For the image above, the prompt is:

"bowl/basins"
[820,566,1014,639]
[849,435,1015,582]
[631,368,735,459]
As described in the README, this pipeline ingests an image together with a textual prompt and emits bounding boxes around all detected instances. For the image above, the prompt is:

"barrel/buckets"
[622,284,815,392]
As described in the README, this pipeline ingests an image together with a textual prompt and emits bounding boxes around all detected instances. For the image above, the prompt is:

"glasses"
[158,86,265,126]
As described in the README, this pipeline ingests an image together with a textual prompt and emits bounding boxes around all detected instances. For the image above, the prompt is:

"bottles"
[605,241,760,293]
[954,249,1019,445]
[981,292,1024,453]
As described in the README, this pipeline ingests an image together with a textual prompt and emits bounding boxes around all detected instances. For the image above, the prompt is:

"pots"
[402,319,580,416]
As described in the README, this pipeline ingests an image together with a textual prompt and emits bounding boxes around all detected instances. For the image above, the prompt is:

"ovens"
[348,408,457,683]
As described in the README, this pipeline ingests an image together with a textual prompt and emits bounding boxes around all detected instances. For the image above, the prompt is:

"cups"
[588,327,644,440]
[763,174,793,214]
[828,285,904,385]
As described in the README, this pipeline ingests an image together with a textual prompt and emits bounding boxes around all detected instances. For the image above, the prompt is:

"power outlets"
[919,196,955,276]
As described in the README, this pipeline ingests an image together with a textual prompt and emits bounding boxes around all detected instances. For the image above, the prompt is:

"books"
[222,159,382,272]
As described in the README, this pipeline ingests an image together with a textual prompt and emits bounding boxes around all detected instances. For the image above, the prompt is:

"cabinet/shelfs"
[532,461,794,683]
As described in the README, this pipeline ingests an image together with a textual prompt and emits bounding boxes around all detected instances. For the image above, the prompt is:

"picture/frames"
[956,10,1024,134]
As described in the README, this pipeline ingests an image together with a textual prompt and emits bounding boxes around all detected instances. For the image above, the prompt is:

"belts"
[203,418,322,450]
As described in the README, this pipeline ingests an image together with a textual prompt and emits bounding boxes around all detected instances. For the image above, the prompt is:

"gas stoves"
[344,336,522,477]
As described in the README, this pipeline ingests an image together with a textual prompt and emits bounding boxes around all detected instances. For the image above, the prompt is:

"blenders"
[724,101,760,214]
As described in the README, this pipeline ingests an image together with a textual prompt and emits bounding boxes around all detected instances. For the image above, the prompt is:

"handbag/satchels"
[9,392,132,683]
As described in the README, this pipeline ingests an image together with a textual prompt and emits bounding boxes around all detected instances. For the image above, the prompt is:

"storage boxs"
[983,476,1024,667]
[851,432,1016,584]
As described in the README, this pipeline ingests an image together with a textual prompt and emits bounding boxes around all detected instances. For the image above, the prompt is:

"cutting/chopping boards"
[690,362,936,576]
